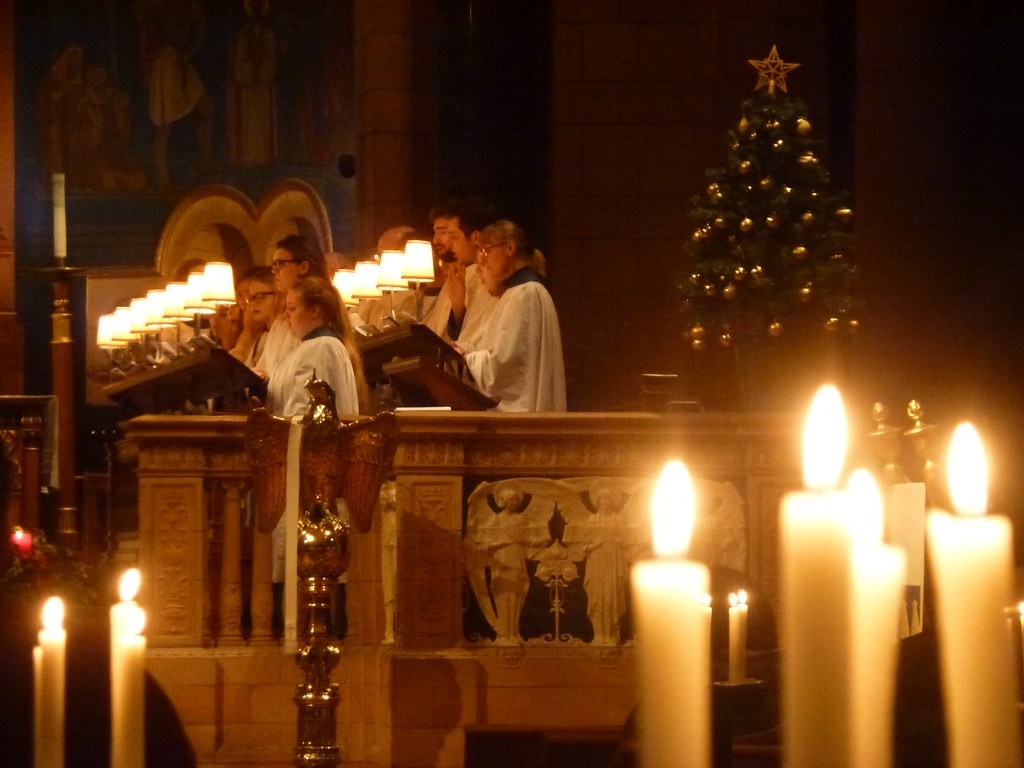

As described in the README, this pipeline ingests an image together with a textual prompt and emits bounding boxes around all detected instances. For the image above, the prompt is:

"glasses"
[271,258,299,268]
[478,241,508,257]
[245,291,277,304]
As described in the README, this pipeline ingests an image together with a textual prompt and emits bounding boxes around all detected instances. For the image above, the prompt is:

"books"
[107,332,263,385]
[356,310,416,337]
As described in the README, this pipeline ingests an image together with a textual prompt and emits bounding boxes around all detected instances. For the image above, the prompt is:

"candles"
[37,597,70,768]
[631,457,711,767]
[738,590,747,681]
[848,464,904,766]
[110,568,140,655]
[33,631,43,768]
[111,608,146,768]
[778,383,861,768]
[1019,602,1024,655]
[729,593,741,682]
[10,530,32,559]
[926,423,1023,768]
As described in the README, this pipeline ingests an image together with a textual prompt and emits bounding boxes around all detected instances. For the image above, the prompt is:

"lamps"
[333,267,359,310]
[184,271,216,348]
[97,314,125,382]
[129,298,161,369]
[163,282,195,356]
[376,251,409,330]
[402,240,436,323]
[111,307,143,374]
[145,290,177,367]
[201,262,237,349]
[353,260,381,336]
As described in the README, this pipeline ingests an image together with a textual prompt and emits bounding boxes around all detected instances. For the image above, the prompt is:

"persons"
[464,477,558,646]
[447,241,547,361]
[552,475,653,649]
[418,202,477,345]
[240,264,286,375]
[249,275,368,421]
[441,217,569,411]
[206,266,270,367]
[244,232,356,383]
[440,204,496,359]
